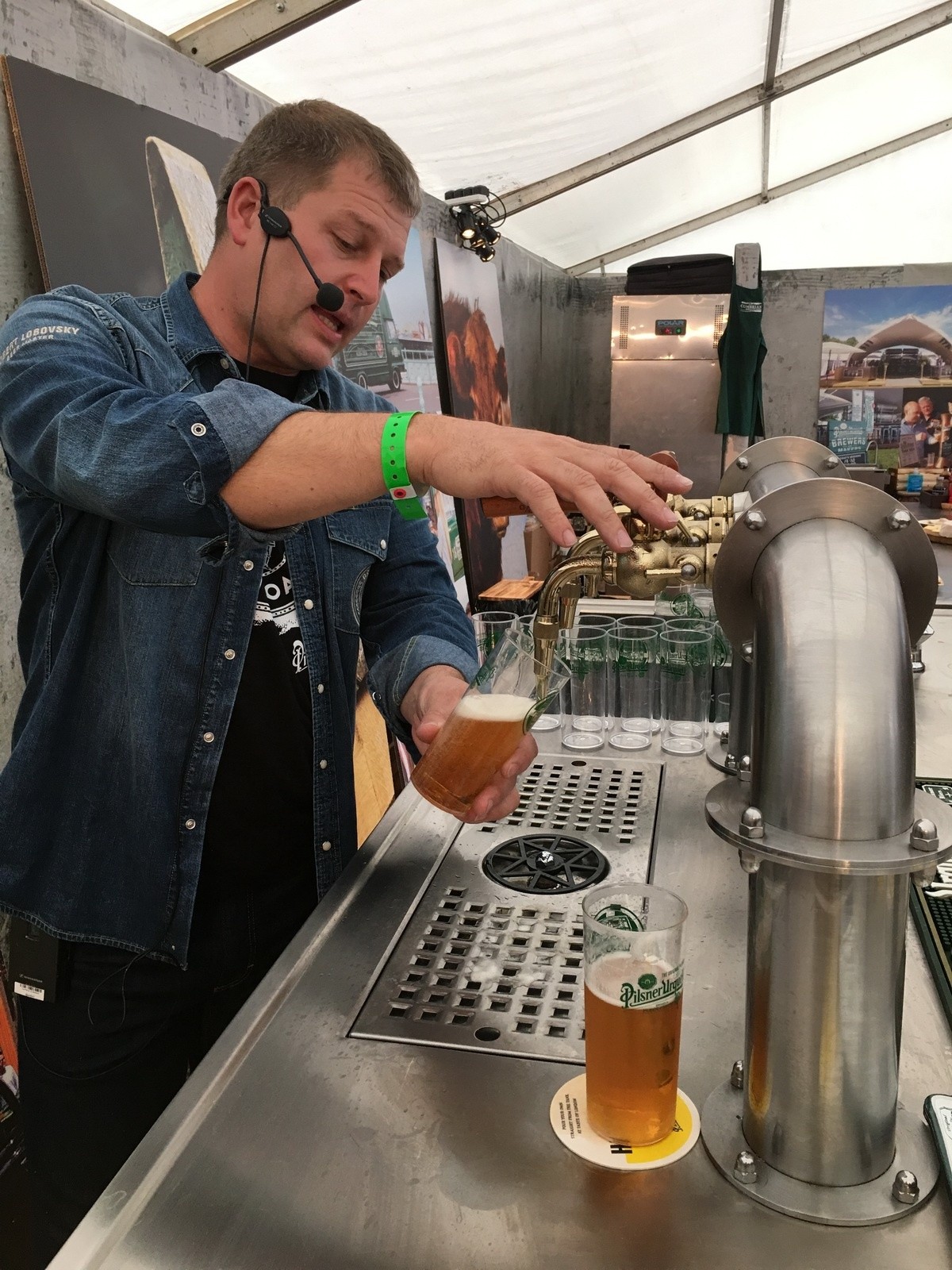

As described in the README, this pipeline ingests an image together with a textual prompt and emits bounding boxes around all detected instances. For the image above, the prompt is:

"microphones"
[289,231,344,312]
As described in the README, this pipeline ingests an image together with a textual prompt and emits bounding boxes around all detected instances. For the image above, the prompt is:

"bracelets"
[380,411,427,521]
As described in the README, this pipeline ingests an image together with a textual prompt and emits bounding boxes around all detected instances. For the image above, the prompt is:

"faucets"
[533,449,747,678]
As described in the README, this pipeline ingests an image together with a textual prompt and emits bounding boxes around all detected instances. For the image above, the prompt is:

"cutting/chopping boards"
[478,576,544,601]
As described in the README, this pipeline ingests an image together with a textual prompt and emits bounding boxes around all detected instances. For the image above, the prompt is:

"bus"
[331,288,408,394]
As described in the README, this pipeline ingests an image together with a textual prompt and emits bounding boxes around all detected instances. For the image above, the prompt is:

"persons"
[0,99,694,1270]
[901,396,939,456]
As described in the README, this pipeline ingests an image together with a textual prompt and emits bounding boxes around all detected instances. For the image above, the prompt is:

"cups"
[927,453,935,469]
[713,619,733,737]
[659,630,713,757]
[410,628,572,817]
[616,616,666,735]
[582,883,689,1146]
[605,627,658,752]
[472,611,518,669]
[664,618,715,739]
[557,628,607,753]
[516,615,562,732]
[654,583,718,618]
[572,614,617,733]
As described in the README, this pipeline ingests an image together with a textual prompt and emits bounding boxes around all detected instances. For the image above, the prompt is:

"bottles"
[934,477,945,490]
[907,468,923,493]
[939,466,951,490]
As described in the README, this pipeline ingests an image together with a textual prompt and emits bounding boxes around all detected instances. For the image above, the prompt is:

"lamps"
[449,204,501,263]
